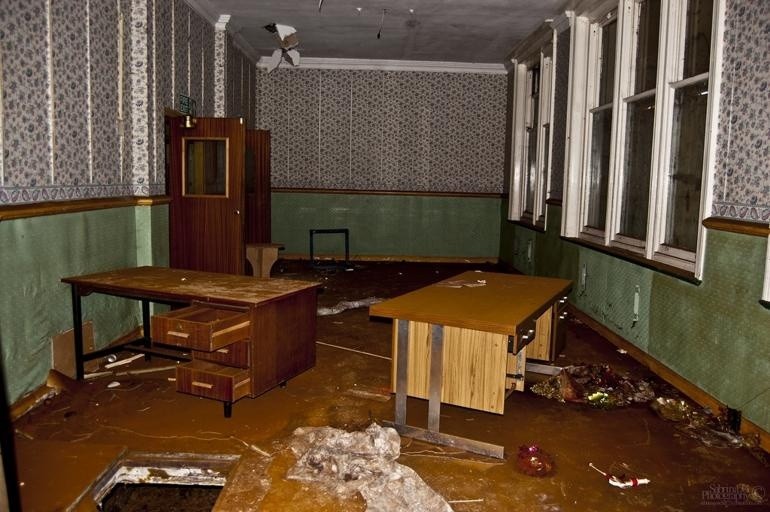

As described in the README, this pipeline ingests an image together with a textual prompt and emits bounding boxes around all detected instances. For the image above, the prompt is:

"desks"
[245,244,285,277]
[61,266,323,418]
[369,270,572,461]
